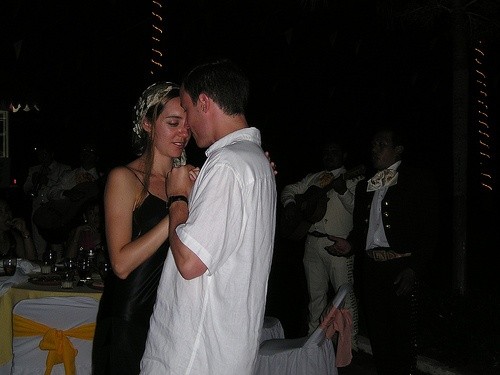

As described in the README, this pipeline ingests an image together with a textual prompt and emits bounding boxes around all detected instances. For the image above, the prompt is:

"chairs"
[9,295,99,375]
[260,316,286,343]
[252,283,350,375]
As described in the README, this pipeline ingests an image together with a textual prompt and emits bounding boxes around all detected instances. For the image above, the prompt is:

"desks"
[0,276,103,375]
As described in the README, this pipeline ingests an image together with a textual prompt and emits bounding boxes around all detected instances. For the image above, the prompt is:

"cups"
[3,249,110,289]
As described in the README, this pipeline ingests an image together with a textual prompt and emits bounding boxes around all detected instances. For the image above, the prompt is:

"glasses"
[372,142,396,150]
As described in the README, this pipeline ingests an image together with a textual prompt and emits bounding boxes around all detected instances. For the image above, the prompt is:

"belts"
[308,231,327,237]
[366,250,412,261]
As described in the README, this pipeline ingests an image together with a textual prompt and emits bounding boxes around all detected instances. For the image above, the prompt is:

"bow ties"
[366,169,398,192]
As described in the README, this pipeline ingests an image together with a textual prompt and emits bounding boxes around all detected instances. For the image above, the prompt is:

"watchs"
[165,194,188,209]
[23,234,30,239]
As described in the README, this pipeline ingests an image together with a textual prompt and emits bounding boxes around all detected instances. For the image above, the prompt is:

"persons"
[0,194,36,260]
[140,61,277,375]
[92,81,279,375]
[66,199,105,259]
[280,127,438,340]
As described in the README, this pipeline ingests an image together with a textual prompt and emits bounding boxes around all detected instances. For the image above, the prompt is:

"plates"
[28,275,78,288]
